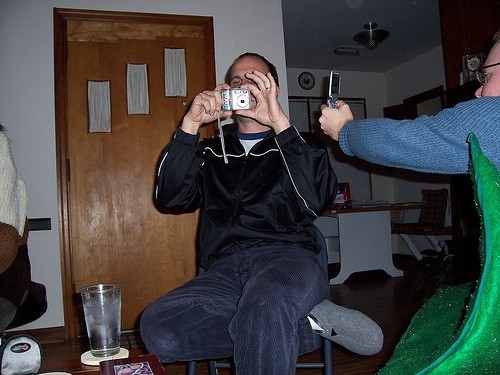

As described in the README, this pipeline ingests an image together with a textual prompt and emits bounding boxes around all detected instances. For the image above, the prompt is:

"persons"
[319,31,500,286]
[139,51,384,375]
[0,124,31,333]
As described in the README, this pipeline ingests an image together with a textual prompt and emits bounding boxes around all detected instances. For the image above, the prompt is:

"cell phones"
[327,70,342,109]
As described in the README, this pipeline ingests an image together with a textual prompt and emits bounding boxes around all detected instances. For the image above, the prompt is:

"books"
[100,354,166,375]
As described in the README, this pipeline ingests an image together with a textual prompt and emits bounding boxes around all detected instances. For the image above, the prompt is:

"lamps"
[352,22,391,50]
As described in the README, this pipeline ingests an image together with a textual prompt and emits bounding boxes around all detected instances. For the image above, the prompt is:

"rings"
[266,88,270,89]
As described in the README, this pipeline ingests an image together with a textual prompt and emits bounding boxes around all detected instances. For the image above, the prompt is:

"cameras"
[221,88,250,111]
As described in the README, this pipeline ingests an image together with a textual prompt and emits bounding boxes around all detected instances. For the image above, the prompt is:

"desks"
[319,204,431,284]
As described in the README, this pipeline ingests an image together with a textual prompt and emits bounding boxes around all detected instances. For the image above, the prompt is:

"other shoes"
[308,298,384,356]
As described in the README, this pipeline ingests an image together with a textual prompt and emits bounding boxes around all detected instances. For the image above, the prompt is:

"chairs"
[188,227,333,375]
[391,188,451,266]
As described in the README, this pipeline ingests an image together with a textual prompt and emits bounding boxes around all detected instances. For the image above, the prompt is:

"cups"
[79,282,122,357]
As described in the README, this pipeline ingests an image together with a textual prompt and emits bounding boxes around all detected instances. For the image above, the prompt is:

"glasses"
[475,63,500,85]
